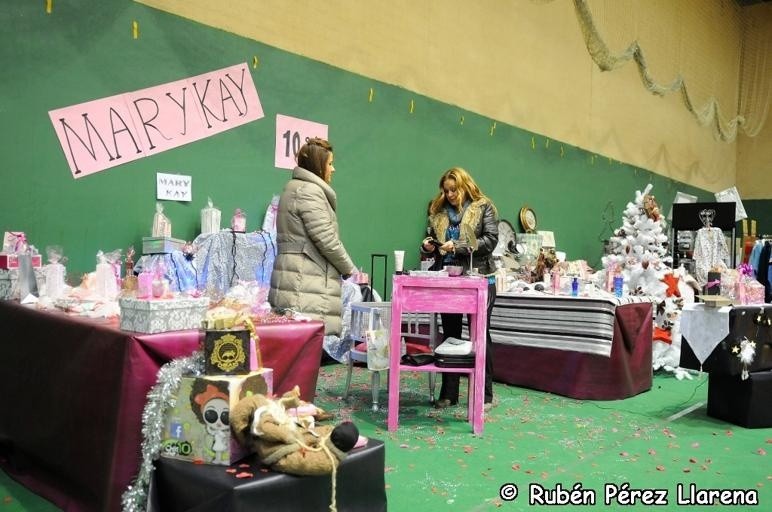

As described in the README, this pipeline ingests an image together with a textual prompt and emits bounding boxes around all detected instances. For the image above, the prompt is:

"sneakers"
[484,402,491,412]
[435,398,459,408]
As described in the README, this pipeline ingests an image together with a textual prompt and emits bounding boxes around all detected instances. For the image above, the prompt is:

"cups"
[614,276,623,298]
[421,261,430,270]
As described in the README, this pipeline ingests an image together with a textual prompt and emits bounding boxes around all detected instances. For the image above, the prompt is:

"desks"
[439,291,655,402]
[386,275,490,438]
[0,288,325,512]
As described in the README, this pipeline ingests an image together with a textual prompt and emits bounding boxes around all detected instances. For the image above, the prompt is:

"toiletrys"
[571,275,578,296]
[394,249,406,275]
[612,266,624,299]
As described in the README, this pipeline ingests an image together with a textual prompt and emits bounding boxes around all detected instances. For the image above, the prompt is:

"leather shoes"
[313,411,333,421]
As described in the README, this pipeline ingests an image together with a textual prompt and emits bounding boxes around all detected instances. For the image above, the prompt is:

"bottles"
[542,266,560,295]
[497,278,503,292]
[571,278,578,296]
[121,258,139,292]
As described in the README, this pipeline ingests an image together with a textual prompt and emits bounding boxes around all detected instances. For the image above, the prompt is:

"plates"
[496,218,516,249]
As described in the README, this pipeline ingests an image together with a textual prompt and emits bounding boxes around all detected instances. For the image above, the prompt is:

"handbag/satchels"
[365,308,390,371]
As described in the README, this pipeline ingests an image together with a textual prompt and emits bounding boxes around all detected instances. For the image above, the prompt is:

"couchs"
[702,306,772,434]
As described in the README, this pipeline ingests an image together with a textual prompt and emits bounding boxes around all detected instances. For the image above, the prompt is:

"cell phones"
[429,240,443,247]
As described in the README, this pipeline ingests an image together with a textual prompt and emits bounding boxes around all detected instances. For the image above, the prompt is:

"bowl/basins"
[443,266,464,277]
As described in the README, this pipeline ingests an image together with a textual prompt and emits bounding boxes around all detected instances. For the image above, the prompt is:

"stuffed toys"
[233,385,361,511]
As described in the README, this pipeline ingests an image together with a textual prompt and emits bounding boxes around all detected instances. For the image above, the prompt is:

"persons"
[264,134,362,423]
[417,166,500,413]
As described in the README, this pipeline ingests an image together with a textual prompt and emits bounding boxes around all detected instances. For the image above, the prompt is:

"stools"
[343,300,390,413]
[149,432,386,511]
